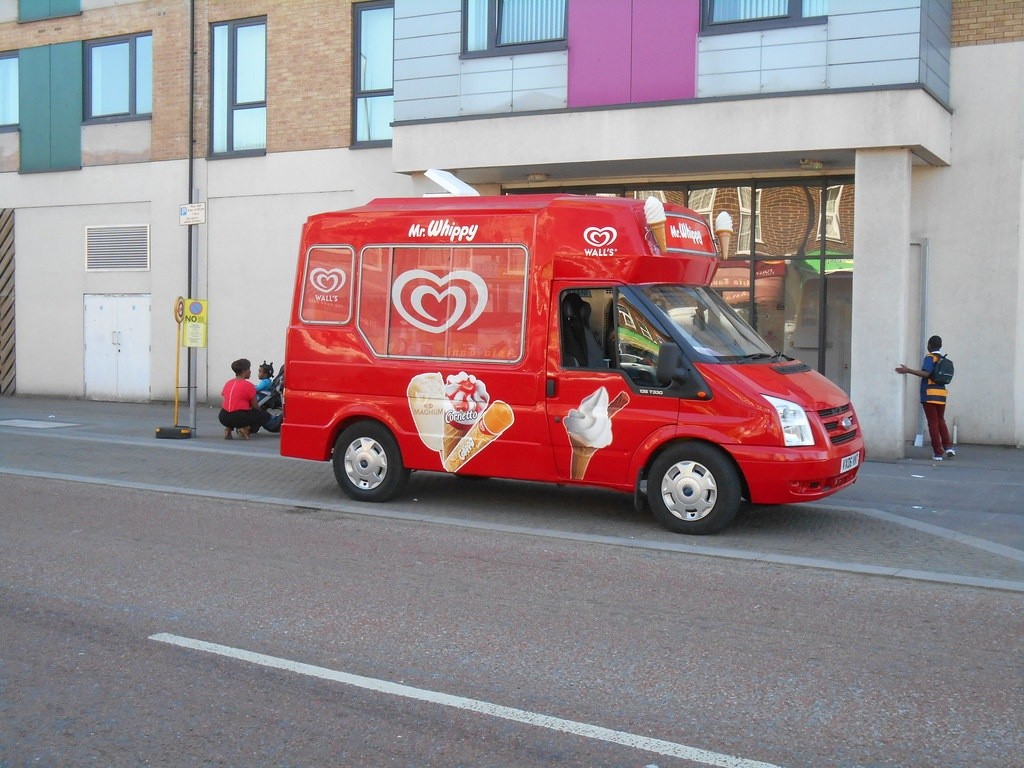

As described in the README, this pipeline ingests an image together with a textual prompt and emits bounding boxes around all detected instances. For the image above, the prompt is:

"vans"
[280,193,866,535]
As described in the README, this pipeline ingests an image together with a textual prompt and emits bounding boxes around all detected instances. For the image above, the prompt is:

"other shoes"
[237,427,250,440]
[224,427,233,440]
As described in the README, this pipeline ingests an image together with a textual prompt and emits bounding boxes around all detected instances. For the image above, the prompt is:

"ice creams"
[405,371,515,472]
[645,195,668,254]
[563,385,630,480]
[715,209,735,259]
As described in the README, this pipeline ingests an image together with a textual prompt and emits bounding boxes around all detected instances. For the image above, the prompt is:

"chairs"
[563,293,605,368]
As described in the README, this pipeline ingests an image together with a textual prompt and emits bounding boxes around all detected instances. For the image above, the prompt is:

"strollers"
[251,365,284,433]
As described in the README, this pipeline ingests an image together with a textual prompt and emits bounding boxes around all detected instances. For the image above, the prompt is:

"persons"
[218,359,263,441]
[254,360,274,402]
[895,336,956,461]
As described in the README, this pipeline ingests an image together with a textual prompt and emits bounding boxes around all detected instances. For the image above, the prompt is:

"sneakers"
[945,448,956,456]
[932,454,943,461]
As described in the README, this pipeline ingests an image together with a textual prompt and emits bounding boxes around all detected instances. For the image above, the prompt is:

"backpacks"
[929,353,954,386]
